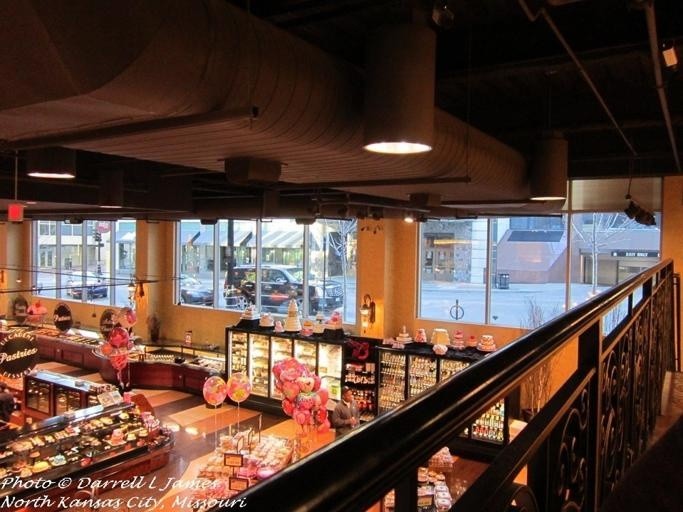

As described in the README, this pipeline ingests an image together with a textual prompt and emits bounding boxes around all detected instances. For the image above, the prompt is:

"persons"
[360,294,375,323]
[330,385,362,439]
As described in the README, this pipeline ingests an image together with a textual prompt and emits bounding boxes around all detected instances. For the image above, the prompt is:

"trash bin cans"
[500,274,510,289]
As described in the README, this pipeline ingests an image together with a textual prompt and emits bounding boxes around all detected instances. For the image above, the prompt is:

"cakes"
[432,344,447,354]
[415,329,426,342]
[300,322,313,336]
[430,329,450,345]
[476,333,495,351]
[466,336,477,346]
[392,343,405,349]
[323,316,344,340]
[285,297,302,331]
[395,326,412,341]
[259,311,273,326]
[241,305,260,320]
[383,338,395,345]
[452,330,464,346]
[275,321,282,331]
[313,315,324,336]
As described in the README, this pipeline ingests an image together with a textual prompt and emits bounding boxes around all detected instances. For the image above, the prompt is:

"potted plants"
[519,295,565,424]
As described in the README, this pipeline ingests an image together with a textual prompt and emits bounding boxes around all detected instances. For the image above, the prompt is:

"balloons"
[99,307,137,372]
[272,358,330,436]
[227,373,252,405]
[202,375,226,408]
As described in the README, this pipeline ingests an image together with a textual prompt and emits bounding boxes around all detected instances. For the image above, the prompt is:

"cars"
[180,273,213,305]
[66,271,108,299]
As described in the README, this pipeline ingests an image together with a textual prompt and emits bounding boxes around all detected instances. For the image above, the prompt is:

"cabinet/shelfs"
[0,401,174,512]
[376,347,436,419]
[223,327,269,411]
[51,382,83,417]
[101,357,215,397]
[343,337,383,423]
[85,392,101,408]
[437,356,506,451]
[318,339,341,419]
[270,335,316,416]
[33,336,101,370]
[24,375,52,419]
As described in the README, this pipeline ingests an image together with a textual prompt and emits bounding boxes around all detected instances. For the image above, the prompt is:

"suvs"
[223,265,344,313]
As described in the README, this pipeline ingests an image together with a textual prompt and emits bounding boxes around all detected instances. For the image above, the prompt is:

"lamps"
[371,207,384,221]
[337,205,350,220]
[24,147,77,179]
[355,205,369,219]
[129,272,144,303]
[360,20,436,155]
[401,211,414,223]
[622,196,657,228]
[359,293,375,332]
[529,137,567,201]
[97,174,123,210]
[6,151,24,222]
